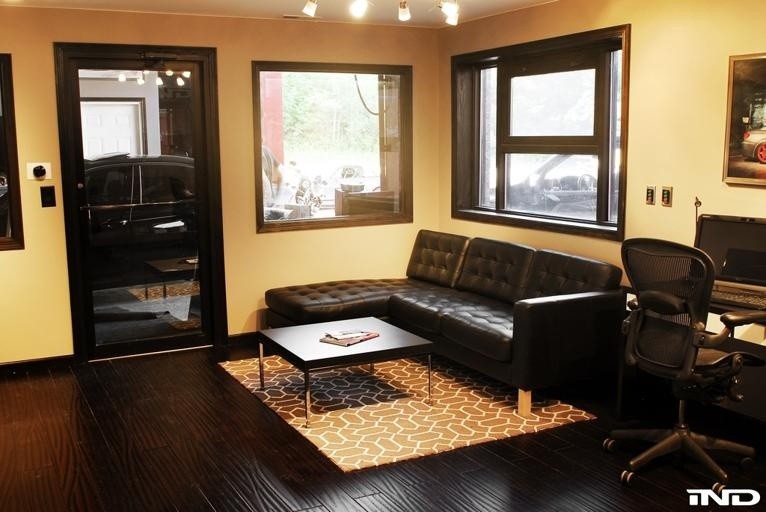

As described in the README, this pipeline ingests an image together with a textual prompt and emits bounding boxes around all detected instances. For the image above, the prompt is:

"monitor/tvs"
[693,215,766,293]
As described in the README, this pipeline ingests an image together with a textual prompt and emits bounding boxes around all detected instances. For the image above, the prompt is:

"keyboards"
[712,289,766,309]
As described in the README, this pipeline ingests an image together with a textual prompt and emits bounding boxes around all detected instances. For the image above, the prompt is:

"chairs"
[599,237,759,494]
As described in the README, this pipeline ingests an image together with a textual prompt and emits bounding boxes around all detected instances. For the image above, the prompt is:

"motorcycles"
[288,160,327,210]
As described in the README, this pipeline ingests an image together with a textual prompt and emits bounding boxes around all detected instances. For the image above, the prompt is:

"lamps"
[301,2,462,27]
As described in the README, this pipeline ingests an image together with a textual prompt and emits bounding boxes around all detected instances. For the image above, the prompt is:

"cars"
[742,120,766,164]
[0,151,285,288]
[488,137,621,223]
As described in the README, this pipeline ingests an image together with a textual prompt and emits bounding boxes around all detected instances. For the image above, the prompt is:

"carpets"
[217,355,598,474]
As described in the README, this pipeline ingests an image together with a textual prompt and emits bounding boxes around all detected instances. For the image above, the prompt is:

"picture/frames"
[721,52,766,187]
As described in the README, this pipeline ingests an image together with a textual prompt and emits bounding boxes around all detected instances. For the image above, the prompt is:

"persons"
[262,145,284,207]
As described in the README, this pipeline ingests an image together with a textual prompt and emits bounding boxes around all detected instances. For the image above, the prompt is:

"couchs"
[267,227,627,417]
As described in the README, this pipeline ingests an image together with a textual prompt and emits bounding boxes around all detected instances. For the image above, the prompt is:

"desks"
[707,278,764,366]
